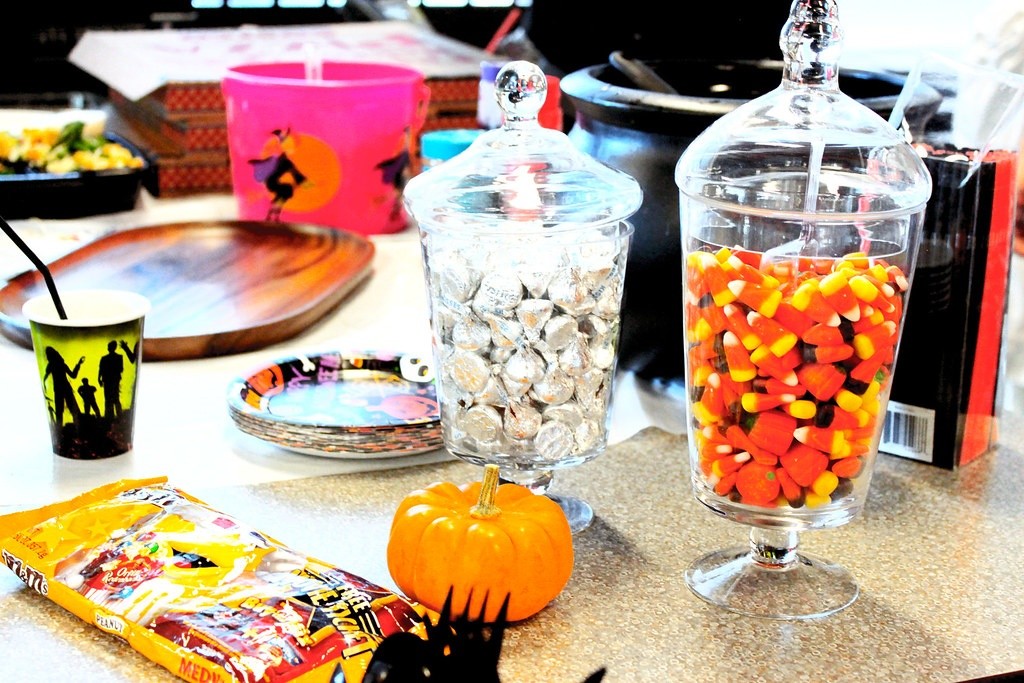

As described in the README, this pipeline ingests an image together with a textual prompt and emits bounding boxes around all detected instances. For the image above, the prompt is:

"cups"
[402,54,642,533]
[671,0,931,628]
[21,287,152,460]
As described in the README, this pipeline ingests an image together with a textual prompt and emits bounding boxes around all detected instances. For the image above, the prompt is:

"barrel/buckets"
[223,59,432,241]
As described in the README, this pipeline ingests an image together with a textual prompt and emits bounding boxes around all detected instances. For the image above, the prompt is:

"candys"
[686,245,908,511]
[435,258,622,462]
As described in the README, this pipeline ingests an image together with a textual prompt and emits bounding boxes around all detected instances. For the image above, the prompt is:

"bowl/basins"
[559,56,944,384]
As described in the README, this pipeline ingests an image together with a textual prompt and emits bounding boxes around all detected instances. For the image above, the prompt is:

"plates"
[228,345,447,459]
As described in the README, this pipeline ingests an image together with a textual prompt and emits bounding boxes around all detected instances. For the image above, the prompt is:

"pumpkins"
[386,464,572,625]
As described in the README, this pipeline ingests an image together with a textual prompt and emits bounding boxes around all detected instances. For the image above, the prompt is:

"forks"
[428,581,514,683]
